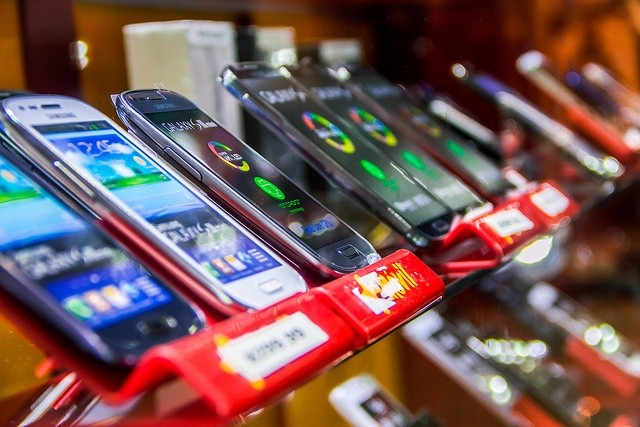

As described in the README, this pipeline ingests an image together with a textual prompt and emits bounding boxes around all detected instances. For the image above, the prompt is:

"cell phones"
[0,133,203,371]
[341,63,510,193]
[328,368,420,426]
[415,90,503,162]
[581,62,639,110]
[278,64,483,216]
[215,61,456,250]
[563,71,619,118]
[0,94,307,317]
[457,71,626,179]
[116,88,378,280]
[522,68,634,163]
